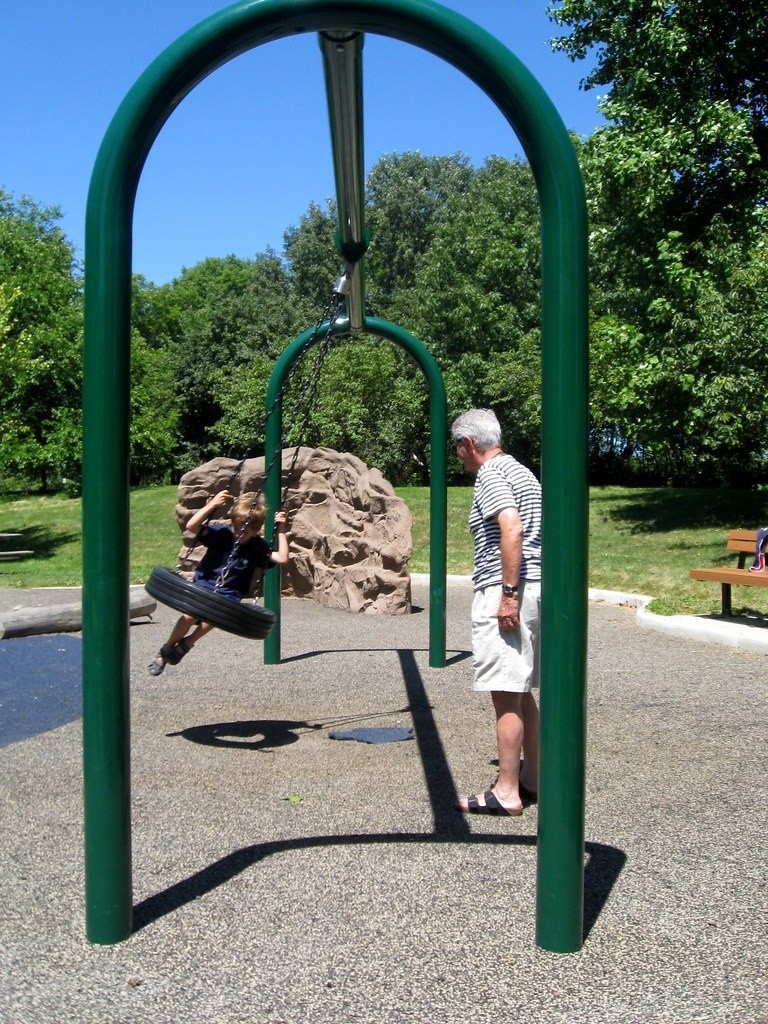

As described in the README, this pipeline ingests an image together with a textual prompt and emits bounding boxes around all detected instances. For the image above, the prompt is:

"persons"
[451,408,543,815]
[148,490,290,676]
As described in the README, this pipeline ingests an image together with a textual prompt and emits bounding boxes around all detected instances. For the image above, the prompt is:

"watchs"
[502,583,519,594]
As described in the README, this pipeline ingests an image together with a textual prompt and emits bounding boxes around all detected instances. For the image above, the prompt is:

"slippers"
[481,774,537,800]
[459,790,513,816]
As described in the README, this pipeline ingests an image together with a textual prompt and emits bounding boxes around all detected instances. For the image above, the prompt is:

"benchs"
[690,529,768,619]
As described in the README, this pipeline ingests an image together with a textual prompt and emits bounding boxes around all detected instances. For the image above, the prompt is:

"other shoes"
[166,637,194,666]
[146,644,170,676]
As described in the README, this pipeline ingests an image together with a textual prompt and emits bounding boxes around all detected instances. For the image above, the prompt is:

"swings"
[145,272,352,640]
